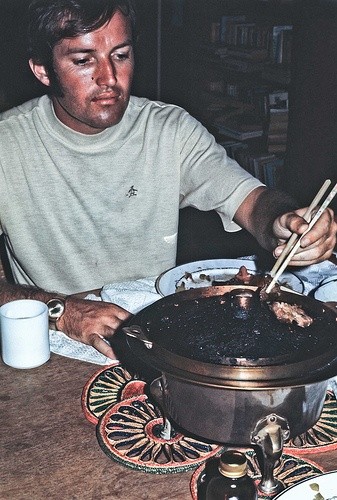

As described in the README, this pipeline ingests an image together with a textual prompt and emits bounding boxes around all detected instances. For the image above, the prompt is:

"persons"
[0,0,337,360]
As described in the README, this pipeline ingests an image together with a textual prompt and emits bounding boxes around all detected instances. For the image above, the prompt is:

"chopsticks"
[257,179,337,303]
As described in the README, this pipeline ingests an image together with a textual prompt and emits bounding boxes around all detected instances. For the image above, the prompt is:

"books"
[187,12,294,190]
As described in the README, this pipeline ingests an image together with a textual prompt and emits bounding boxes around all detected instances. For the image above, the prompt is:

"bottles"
[205,449,259,500]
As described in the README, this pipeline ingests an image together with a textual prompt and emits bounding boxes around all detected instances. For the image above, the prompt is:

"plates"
[273,469,337,500]
[155,257,305,295]
[314,278,337,307]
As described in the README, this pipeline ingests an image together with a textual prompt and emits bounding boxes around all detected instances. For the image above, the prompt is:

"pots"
[112,285,337,445]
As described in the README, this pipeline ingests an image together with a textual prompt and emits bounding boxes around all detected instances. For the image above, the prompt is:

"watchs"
[46,294,69,332]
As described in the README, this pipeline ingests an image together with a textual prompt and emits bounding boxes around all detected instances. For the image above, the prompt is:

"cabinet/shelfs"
[199,0,337,214]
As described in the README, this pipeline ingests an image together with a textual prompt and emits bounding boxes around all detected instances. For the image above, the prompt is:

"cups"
[0,297,64,369]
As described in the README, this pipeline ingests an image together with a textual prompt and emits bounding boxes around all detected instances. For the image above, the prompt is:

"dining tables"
[0,251,337,500]
